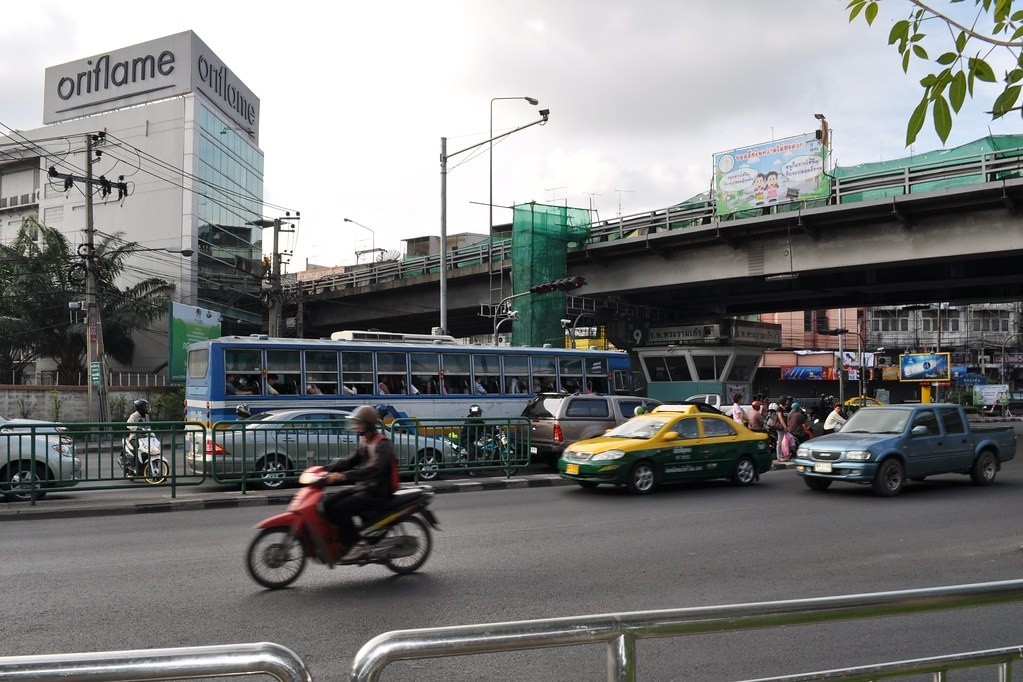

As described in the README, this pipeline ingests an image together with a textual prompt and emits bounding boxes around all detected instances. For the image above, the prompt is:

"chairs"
[387,376,395,393]
[431,378,439,393]
[399,378,407,393]
[574,379,581,393]
[493,379,500,393]
[462,378,471,393]
[533,376,541,393]
[546,382,554,391]
[518,377,528,393]
[290,377,300,394]
[253,380,262,394]
[506,377,517,393]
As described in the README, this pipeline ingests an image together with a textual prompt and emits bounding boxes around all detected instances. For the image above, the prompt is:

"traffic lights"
[552,276,585,291]
[817,329,850,336]
[529,279,556,295]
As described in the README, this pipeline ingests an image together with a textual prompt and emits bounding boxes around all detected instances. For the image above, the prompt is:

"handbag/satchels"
[781,432,795,457]
[774,417,786,431]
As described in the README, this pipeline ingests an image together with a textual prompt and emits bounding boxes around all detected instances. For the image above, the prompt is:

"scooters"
[117,418,169,484]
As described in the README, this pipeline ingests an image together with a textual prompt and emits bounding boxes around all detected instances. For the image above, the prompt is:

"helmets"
[344,405,380,424]
[752,401,761,410]
[134,399,152,415]
[791,402,799,410]
[236,403,251,418]
[821,394,834,402]
[634,406,644,416]
[470,405,483,414]
[768,403,778,410]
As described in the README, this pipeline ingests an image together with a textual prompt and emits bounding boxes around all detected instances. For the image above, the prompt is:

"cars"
[186,408,456,490]
[0,414,82,502]
[844,395,885,413]
[978,390,1023,417]
[557,405,772,494]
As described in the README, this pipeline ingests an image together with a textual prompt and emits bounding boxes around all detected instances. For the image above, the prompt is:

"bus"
[184,330,646,445]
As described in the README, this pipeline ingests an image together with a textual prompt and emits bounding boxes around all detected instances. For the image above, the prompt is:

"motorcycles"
[243,455,445,590]
[744,402,849,461]
[439,425,519,476]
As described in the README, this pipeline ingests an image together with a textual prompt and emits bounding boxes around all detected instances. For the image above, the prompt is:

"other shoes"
[777,457,790,462]
[341,541,371,560]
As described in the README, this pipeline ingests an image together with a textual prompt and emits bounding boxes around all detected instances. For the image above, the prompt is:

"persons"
[321,406,400,559]
[819,394,833,418]
[127,399,151,471]
[732,393,814,462]
[227,374,593,396]
[634,406,648,416]
[461,404,500,476]
[823,403,847,435]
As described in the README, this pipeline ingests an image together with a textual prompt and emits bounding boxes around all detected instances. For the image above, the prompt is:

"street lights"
[344,218,375,263]
[83,131,195,441]
[439,109,550,336]
[489,97,538,315]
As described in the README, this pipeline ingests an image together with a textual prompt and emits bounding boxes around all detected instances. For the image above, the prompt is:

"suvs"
[511,389,665,470]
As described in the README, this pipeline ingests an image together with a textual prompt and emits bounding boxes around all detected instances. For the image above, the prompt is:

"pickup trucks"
[794,403,1016,496]
